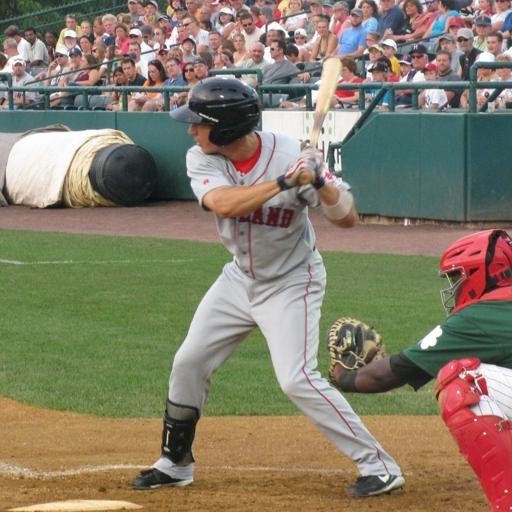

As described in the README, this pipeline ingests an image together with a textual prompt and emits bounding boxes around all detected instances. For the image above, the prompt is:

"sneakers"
[131,456,195,491]
[347,468,406,497]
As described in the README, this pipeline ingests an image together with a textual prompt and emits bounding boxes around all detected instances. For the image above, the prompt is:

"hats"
[11,0,492,74]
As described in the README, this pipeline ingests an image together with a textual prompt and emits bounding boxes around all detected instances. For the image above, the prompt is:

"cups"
[487,102,494,113]
[156,100,163,113]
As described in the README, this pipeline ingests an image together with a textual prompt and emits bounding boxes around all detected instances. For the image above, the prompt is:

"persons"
[132,77,405,498]
[329,228,511,509]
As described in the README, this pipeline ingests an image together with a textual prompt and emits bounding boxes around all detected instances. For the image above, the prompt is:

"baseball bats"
[299,58,342,184]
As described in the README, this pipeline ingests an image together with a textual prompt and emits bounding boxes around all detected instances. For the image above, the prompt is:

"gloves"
[276,154,322,191]
[302,145,327,190]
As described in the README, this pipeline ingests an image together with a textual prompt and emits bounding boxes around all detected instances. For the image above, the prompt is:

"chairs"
[263,41,439,106]
[50,95,114,110]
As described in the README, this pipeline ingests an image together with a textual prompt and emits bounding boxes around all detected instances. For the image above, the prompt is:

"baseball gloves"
[328,316,383,384]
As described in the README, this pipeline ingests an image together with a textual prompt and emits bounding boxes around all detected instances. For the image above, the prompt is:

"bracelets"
[275,176,292,191]
[313,178,326,190]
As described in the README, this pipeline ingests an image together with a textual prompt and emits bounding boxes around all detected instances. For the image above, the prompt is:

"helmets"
[437,227,512,318]
[169,76,262,145]
